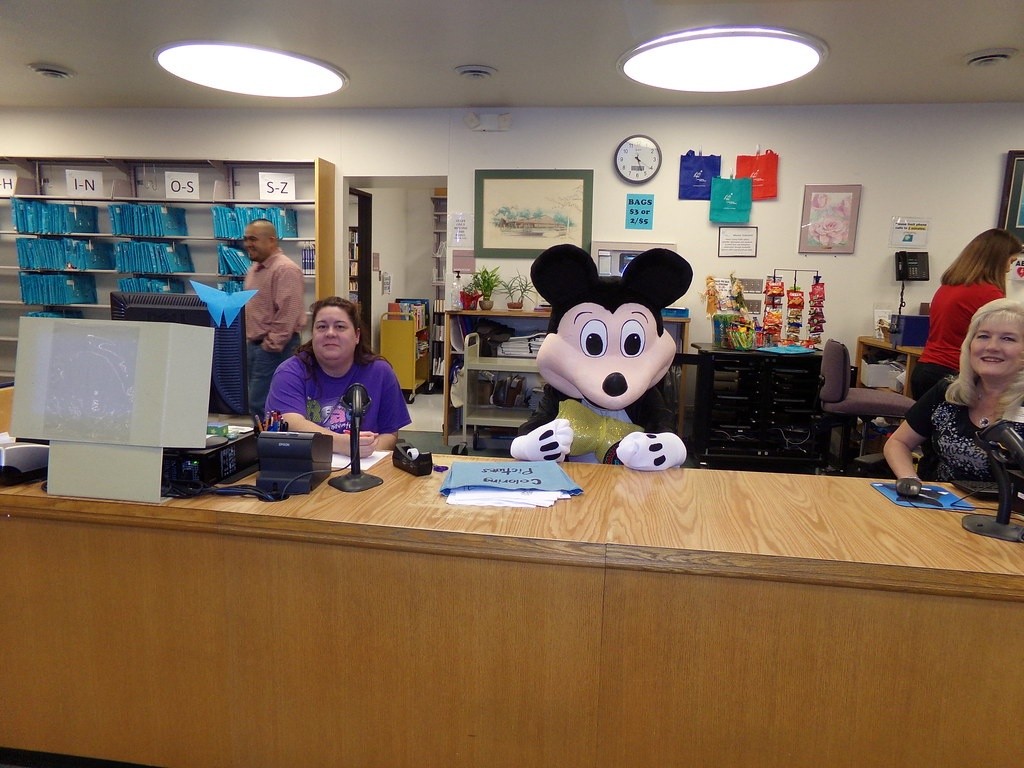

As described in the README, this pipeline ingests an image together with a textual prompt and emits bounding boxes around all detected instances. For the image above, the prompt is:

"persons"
[243,219,305,419]
[884,297,1024,482]
[909,227,1024,482]
[265,296,413,458]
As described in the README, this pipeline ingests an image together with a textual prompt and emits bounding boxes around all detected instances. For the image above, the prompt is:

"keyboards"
[951,479,1001,500]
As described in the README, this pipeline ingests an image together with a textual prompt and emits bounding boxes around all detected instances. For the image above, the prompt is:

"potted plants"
[470,264,501,309]
[498,270,536,308]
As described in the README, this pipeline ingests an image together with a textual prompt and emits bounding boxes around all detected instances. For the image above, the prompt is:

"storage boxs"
[888,314,929,347]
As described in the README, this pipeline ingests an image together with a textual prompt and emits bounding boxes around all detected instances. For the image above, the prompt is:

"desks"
[0,451,1024,767]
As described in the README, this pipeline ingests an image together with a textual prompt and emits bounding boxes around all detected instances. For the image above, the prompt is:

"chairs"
[810,339,918,475]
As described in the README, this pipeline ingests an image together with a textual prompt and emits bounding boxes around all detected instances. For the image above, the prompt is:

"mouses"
[896,477,922,496]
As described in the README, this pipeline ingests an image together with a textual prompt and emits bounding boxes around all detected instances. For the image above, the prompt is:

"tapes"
[406,448,419,460]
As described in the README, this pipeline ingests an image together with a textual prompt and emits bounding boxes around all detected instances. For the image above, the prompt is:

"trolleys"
[451,332,546,457]
[379,312,433,404]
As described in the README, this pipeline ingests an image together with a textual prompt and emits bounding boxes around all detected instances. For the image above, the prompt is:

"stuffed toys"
[511,244,694,470]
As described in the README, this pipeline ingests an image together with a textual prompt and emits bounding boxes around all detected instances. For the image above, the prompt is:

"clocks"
[613,134,662,184]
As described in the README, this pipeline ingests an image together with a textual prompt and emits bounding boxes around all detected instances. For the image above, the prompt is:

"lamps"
[150,39,350,98]
[614,23,830,94]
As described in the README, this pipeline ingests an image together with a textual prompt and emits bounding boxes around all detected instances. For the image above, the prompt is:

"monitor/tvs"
[109,292,252,448]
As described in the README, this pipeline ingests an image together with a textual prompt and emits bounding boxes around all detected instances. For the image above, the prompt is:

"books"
[433,357,445,375]
[500,332,544,355]
[432,299,445,312]
[432,324,444,342]
[388,302,425,331]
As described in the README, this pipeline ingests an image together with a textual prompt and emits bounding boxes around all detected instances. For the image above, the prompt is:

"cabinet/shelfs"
[854,334,925,452]
[688,342,827,474]
[461,333,539,447]
[2,153,337,380]
[348,227,360,307]
[379,312,433,404]
[441,309,691,448]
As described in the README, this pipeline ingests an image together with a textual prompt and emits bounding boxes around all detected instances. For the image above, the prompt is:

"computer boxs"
[162,429,260,492]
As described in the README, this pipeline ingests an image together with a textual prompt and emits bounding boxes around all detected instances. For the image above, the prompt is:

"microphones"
[341,382,369,430]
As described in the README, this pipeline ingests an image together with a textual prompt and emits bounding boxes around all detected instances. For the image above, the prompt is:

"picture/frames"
[475,169,593,258]
[799,184,862,254]
[997,149,1024,253]
